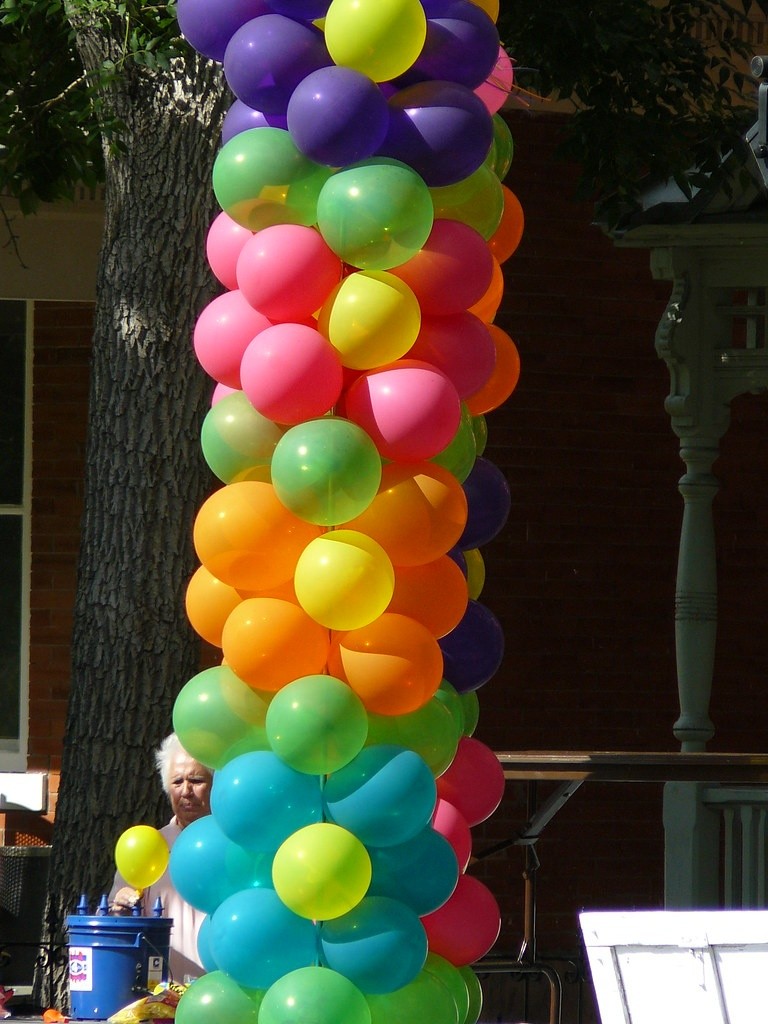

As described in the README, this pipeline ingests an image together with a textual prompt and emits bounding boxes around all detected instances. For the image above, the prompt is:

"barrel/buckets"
[63,915,175,1022]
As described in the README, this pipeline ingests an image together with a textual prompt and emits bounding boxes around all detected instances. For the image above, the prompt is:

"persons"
[96,730,216,984]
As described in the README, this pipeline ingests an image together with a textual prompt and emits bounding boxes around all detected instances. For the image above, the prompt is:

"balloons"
[168,0,526,1024]
[43,1009,69,1023]
[115,826,169,895]
